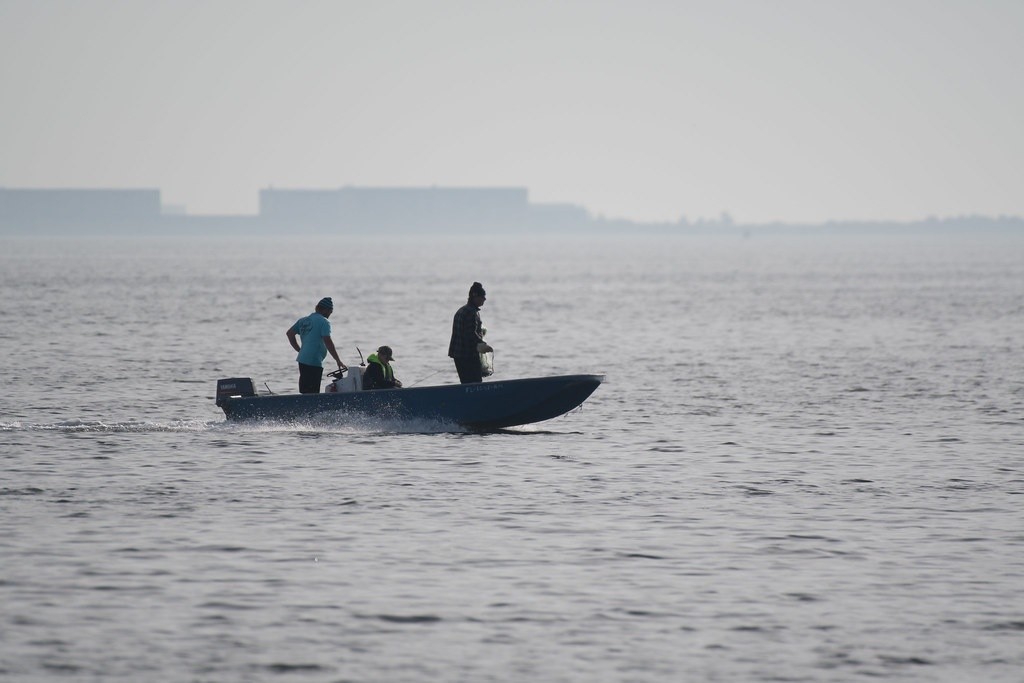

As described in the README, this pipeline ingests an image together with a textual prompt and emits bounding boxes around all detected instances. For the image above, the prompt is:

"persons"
[364,345,403,389]
[285,296,347,393]
[448,281,493,384]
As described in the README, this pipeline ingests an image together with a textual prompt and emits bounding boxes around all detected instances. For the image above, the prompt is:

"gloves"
[477,342,493,354]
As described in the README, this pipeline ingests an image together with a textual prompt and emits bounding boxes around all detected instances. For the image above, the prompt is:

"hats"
[376,346,395,361]
[469,282,486,300]
[318,297,333,311]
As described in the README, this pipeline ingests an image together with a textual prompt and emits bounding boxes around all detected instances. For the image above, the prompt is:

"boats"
[215,373,603,431]
